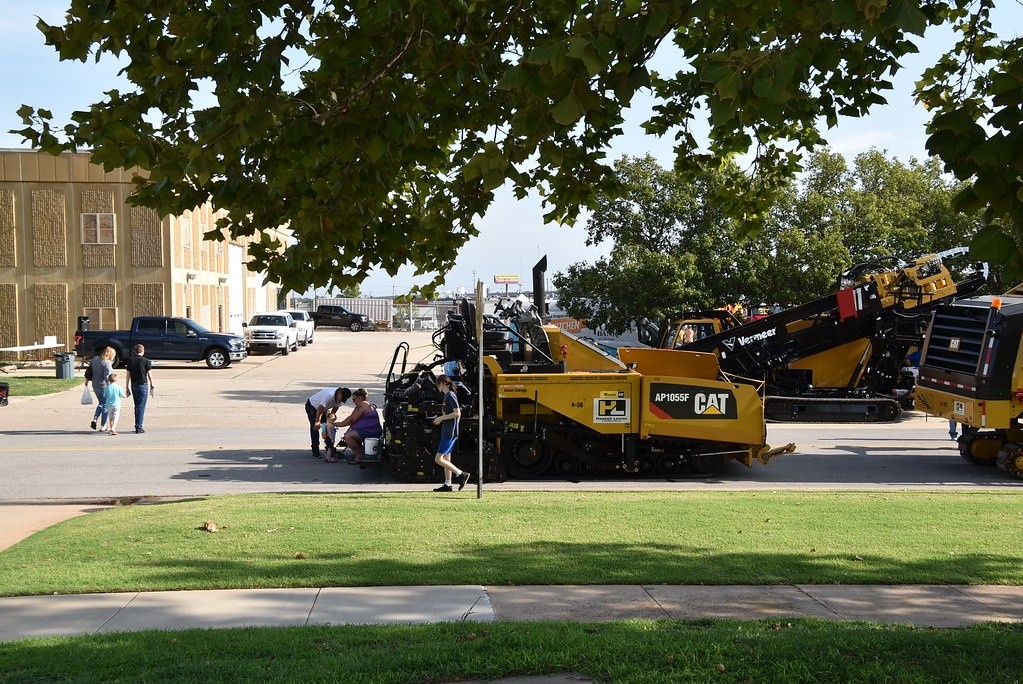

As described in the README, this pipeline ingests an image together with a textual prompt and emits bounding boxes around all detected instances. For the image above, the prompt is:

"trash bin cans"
[53,352,75,379]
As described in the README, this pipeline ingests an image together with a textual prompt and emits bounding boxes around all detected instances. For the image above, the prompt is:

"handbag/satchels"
[81,386,93,404]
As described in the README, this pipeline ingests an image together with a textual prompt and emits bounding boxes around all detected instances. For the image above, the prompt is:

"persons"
[324,413,337,463]
[676,324,694,344]
[103,374,127,435]
[305,387,351,459]
[433,375,470,492]
[85,346,117,432]
[125,344,154,433]
[333,388,382,465]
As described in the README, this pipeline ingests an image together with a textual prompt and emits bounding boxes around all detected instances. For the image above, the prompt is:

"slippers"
[348,459,360,464]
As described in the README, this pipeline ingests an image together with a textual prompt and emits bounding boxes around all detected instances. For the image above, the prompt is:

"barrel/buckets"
[365,438,379,455]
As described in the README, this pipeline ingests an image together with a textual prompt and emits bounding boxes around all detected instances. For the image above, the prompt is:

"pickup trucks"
[308,305,369,332]
[74,315,248,370]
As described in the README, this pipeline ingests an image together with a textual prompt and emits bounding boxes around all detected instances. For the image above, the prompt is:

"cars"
[362,320,374,331]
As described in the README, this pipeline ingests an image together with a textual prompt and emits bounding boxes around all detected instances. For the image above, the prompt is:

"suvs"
[241,312,299,356]
[276,309,315,346]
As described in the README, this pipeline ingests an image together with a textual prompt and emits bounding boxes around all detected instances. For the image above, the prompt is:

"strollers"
[0,369,10,406]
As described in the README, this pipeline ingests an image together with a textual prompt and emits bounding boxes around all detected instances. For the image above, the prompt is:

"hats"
[341,388,351,403]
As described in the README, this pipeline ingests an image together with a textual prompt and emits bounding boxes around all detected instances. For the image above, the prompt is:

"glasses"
[352,396,359,401]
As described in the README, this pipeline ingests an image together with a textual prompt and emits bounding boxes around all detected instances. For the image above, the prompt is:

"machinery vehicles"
[911,280,1023,484]
[655,299,925,425]
[375,242,990,481]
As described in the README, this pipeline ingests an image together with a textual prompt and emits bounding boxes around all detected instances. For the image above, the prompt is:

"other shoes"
[110,429,117,435]
[100,427,107,432]
[136,429,145,433]
[90,421,96,430]
[458,472,470,490]
[433,484,452,492]
[326,458,336,462]
[313,455,321,458]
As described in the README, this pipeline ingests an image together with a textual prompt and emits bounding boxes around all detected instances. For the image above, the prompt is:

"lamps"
[189,274,198,280]
[221,278,229,283]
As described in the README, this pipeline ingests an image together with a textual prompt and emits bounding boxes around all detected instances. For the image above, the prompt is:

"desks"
[0,343,65,368]
